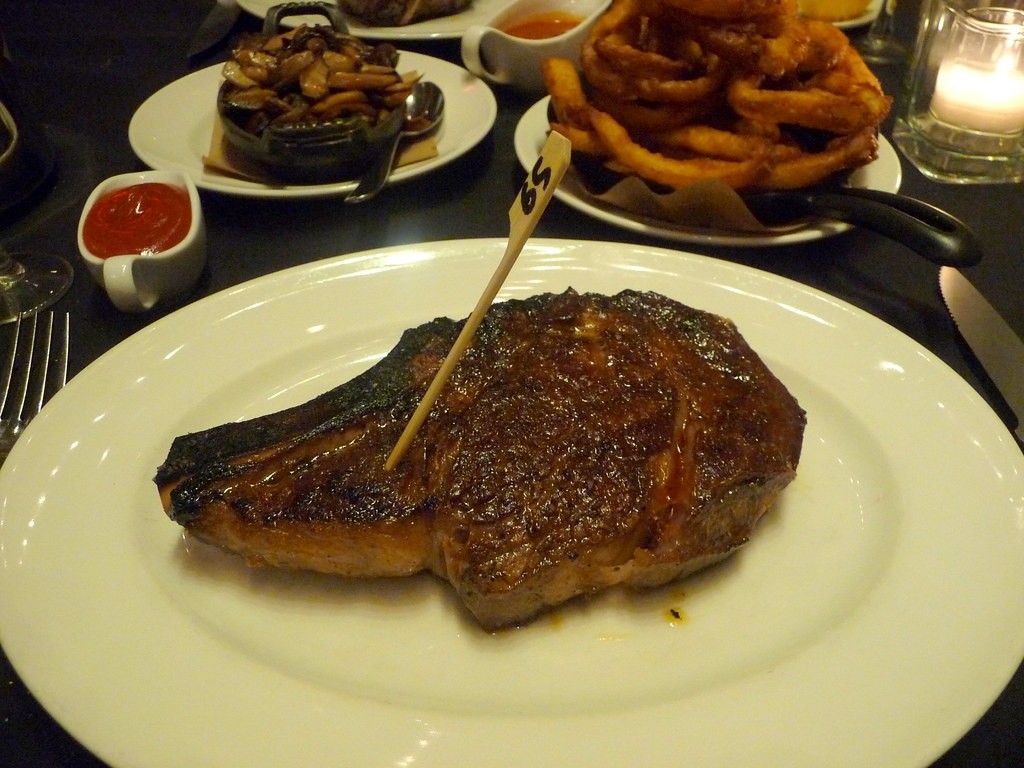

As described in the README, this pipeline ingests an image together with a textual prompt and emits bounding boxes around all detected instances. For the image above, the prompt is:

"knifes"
[939,266,1024,444]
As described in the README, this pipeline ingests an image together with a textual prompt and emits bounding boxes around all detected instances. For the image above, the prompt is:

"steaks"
[150,287,807,632]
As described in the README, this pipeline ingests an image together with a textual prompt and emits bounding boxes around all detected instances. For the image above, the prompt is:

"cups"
[76,168,206,316]
[460,0,615,93]
[890,0,1024,185]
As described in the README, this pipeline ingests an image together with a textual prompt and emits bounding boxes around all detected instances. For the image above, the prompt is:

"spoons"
[344,82,443,203]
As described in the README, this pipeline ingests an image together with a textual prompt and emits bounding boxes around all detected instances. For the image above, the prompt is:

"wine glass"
[0,101,75,327]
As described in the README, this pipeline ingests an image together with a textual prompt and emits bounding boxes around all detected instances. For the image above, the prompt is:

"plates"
[233,0,498,38]
[127,48,498,198]
[1,236,1023,768]
[514,92,904,246]
[829,0,886,30]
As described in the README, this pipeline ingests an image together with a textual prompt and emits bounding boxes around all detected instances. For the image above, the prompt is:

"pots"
[218,1,406,177]
[549,98,985,270]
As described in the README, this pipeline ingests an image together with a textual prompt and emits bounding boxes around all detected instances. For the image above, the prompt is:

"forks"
[0,310,71,470]
